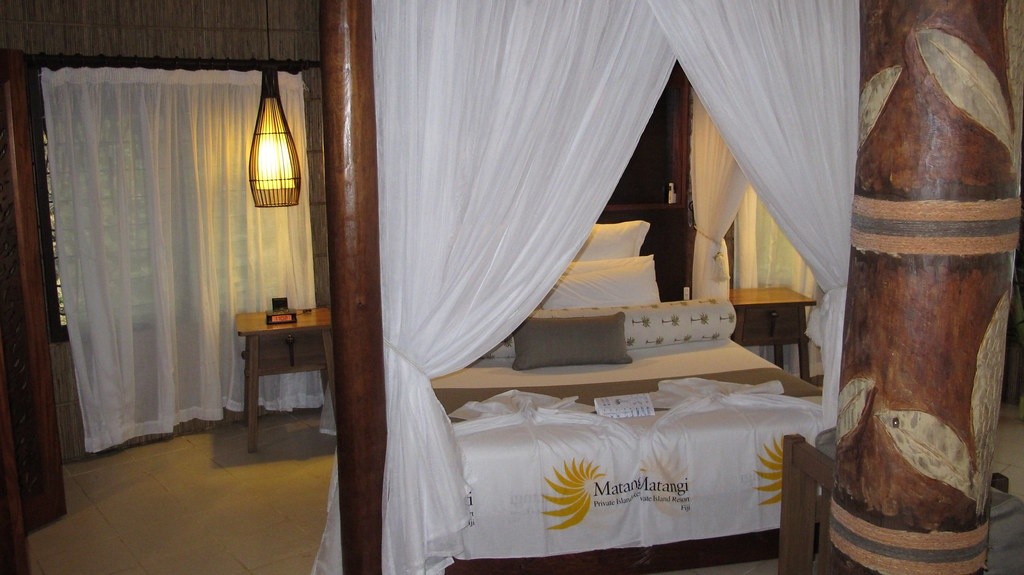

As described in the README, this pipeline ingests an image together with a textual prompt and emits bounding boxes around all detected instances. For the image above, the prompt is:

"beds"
[428,335,826,575]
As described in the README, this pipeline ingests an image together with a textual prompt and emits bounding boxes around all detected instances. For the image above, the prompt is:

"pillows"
[512,312,632,371]
[477,298,736,359]
[571,220,651,262]
[540,253,659,310]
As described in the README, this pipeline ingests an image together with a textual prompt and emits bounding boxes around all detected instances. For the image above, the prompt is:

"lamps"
[247,0,301,208]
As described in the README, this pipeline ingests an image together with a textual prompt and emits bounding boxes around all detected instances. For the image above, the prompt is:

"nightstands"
[234,308,339,454]
[727,285,819,381]
